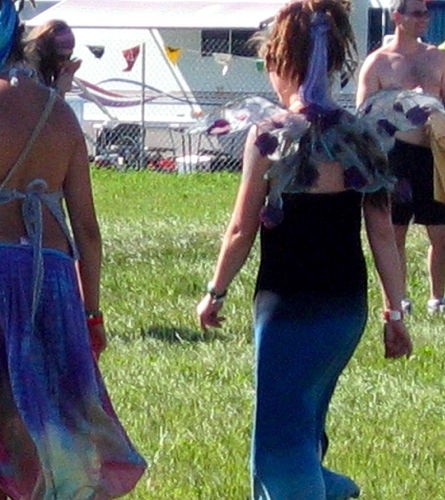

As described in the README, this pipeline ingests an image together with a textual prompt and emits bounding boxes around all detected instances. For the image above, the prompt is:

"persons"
[196,2,414,499]
[355,0,445,317]
[21,17,82,99]
[0,0,151,500]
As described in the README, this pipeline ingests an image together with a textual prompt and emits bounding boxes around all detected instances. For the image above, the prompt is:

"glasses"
[55,54,71,62]
[413,10,432,18]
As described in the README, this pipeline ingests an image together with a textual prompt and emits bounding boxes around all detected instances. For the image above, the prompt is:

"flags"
[86,44,106,59]
[213,51,234,75]
[165,46,184,64]
[122,44,140,71]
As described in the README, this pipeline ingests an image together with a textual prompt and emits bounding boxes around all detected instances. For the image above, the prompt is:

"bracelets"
[383,308,404,320]
[206,281,229,300]
[85,310,104,325]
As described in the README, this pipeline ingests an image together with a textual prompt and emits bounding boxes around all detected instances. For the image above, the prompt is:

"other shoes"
[426,302,445,318]
